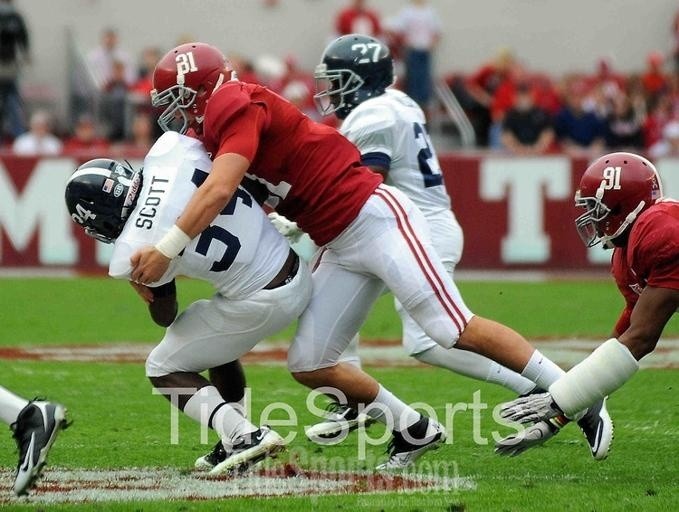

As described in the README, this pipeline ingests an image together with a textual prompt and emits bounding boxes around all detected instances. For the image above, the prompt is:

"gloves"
[501,394,562,422]
[494,414,572,457]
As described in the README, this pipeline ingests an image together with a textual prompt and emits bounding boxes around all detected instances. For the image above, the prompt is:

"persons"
[0,384,73,496]
[128,44,615,469]
[2,0,678,165]
[307,34,546,438]
[494,152,679,458]
[66,130,311,472]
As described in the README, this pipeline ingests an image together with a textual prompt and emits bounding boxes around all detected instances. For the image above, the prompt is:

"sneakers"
[307,405,374,441]
[196,426,283,479]
[577,395,614,461]
[10,396,68,496]
[378,414,448,470]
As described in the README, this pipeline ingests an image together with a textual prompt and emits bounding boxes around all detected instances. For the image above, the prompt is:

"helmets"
[313,34,395,118]
[575,153,661,249]
[151,43,232,133]
[65,157,142,243]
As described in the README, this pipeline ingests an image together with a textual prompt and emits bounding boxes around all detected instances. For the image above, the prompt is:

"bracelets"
[155,224,191,261]
